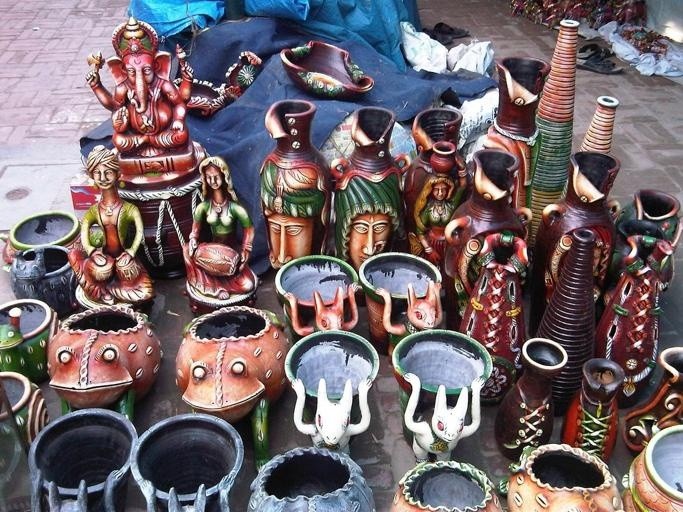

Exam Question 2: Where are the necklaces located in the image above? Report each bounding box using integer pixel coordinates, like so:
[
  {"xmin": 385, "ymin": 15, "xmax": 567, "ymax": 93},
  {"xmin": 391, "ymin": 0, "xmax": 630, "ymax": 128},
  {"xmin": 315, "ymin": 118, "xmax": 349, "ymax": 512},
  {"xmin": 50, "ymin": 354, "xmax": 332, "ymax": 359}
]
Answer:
[
  {"xmin": 100, "ymin": 199, "xmax": 120, "ymax": 216},
  {"xmin": 212, "ymin": 199, "xmax": 228, "ymax": 214}
]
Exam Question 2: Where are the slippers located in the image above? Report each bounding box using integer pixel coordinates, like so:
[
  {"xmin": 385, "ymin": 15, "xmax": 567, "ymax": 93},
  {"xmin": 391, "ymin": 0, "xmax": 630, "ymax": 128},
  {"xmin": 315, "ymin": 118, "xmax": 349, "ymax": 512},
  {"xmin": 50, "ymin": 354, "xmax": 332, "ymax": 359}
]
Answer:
[
  {"xmin": 422, "ymin": 22, "xmax": 470, "ymax": 45},
  {"xmin": 577, "ymin": 43, "xmax": 613, "ymax": 59},
  {"xmin": 575, "ymin": 55, "xmax": 623, "ymax": 75}
]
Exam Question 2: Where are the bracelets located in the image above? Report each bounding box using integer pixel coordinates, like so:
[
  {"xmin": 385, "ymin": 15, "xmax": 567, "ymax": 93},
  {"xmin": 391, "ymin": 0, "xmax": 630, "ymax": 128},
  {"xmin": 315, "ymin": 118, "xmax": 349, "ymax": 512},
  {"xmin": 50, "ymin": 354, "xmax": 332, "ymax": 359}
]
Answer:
[
  {"xmin": 457, "ymin": 167, "xmax": 467, "ymax": 177},
  {"xmin": 425, "ymin": 247, "xmax": 433, "ymax": 254}
]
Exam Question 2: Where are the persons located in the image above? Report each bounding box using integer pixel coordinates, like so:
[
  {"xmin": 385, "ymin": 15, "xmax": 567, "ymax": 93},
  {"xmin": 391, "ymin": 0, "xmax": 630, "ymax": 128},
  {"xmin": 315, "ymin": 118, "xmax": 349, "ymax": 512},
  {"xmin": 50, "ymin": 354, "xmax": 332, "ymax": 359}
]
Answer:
[
  {"xmin": 334, "ymin": 166, "xmax": 404, "ymax": 272},
  {"xmin": 413, "ymin": 154, "xmax": 467, "ymax": 298},
  {"xmin": 260, "ymin": 160, "xmax": 325, "ymax": 270},
  {"xmin": 68, "ymin": 145, "xmax": 155, "ymax": 306},
  {"xmin": 183, "ymin": 156, "xmax": 255, "ymax": 300}
]
